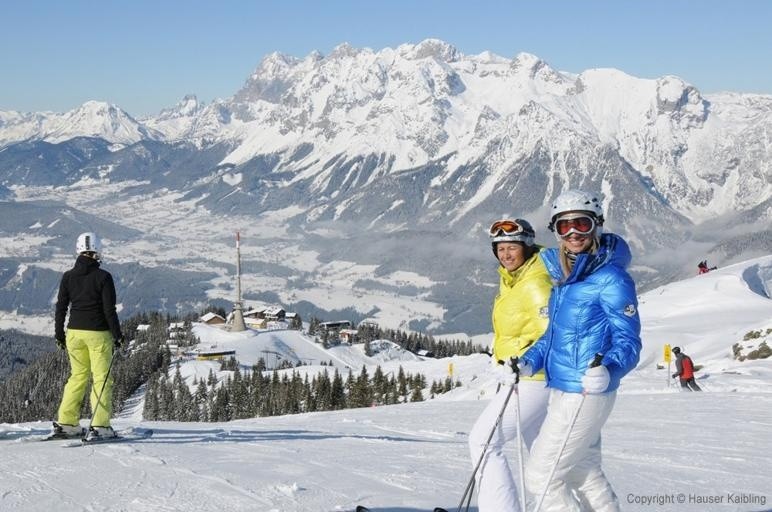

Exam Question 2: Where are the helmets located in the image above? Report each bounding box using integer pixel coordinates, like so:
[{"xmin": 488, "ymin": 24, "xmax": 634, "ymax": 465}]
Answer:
[
  {"xmin": 672, "ymin": 347, "xmax": 680, "ymax": 354},
  {"xmin": 76, "ymin": 231, "xmax": 101, "ymax": 257},
  {"xmin": 491, "ymin": 216, "xmax": 535, "ymax": 248},
  {"xmin": 549, "ymin": 188, "xmax": 603, "ymax": 225}
]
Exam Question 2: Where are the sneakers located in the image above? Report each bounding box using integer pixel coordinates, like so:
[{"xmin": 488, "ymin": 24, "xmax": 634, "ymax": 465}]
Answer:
[
  {"xmin": 58, "ymin": 423, "xmax": 83, "ymax": 437},
  {"xmin": 91, "ymin": 425, "xmax": 114, "ymax": 438}
]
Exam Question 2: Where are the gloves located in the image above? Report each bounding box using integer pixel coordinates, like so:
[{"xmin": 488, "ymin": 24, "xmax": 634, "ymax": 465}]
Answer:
[
  {"xmin": 505, "ymin": 359, "xmax": 532, "ymax": 384},
  {"xmin": 115, "ymin": 336, "xmax": 124, "ymax": 353},
  {"xmin": 581, "ymin": 365, "xmax": 612, "ymax": 394},
  {"xmin": 55, "ymin": 339, "xmax": 65, "ymax": 352}
]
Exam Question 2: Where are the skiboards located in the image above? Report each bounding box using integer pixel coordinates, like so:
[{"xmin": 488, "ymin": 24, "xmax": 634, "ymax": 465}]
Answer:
[{"xmin": 13, "ymin": 426, "xmax": 153, "ymax": 448}]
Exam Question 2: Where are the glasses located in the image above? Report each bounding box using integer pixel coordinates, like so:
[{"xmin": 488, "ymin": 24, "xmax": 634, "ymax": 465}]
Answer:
[
  {"xmin": 489, "ymin": 220, "xmax": 534, "ymax": 238},
  {"xmin": 549, "ymin": 215, "xmax": 603, "ymax": 239}
]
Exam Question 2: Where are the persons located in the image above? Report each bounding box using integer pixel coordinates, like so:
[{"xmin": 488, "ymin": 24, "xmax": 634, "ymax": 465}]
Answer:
[
  {"xmin": 467, "ymin": 219, "xmax": 566, "ymax": 510},
  {"xmin": 55, "ymin": 232, "xmax": 122, "ymax": 440},
  {"xmin": 505, "ymin": 189, "xmax": 640, "ymax": 512},
  {"xmin": 671, "ymin": 347, "xmax": 703, "ymax": 391},
  {"xmin": 703, "ymin": 260, "xmax": 708, "ymax": 274},
  {"xmin": 699, "ymin": 261, "xmax": 707, "ymax": 274}
]
